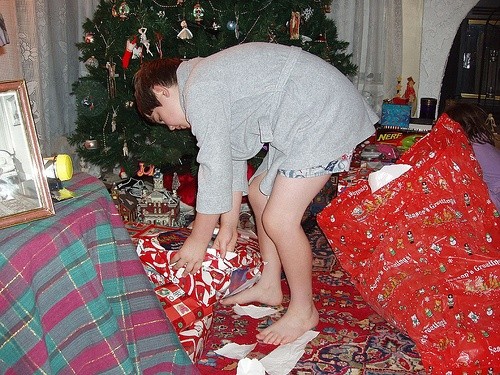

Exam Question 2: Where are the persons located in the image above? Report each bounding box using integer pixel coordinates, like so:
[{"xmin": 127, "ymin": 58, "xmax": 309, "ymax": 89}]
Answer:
[
  {"xmin": 132, "ymin": 41, "xmax": 380, "ymax": 346},
  {"xmin": 445, "ymin": 103, "xmax": 500, "ymax": 213}
]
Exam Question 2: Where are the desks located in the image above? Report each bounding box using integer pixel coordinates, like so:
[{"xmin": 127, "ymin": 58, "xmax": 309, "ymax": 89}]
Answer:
[{"xmin": 0, "ymin": 168, "xmax": 200, "ymax": 375}]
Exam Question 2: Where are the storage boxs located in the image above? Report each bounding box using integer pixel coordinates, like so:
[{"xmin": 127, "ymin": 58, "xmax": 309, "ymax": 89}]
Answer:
[{"xmin": 381, "ymin": 103, "xmax": 411, "ymax": 128}]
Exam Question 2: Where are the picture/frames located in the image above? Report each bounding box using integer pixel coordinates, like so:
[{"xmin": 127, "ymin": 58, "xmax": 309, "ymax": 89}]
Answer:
[{"xmin": 0, "ymin": 78, "xmax": 56, "ymax": 230}]
